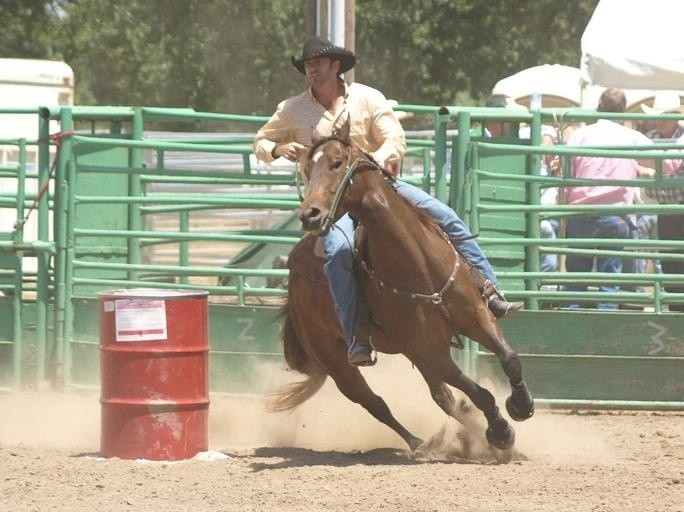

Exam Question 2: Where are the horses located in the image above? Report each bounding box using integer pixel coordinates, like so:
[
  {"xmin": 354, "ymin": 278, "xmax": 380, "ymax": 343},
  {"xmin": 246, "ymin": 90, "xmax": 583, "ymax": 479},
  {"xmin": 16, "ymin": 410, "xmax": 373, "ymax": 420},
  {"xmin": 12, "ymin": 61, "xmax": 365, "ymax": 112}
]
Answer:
[{"xmin": 256, "ymin": 110, "xmax": 535, "ymax": 464}]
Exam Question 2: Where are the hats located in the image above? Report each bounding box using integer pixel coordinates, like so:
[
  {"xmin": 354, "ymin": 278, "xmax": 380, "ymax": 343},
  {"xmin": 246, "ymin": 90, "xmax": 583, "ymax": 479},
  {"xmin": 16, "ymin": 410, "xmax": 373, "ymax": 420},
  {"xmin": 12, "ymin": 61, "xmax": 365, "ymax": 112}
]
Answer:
[
  {"xmin": 641, "ymin": 90, "xmax": 684, "ymax": 113},
  {"xmin": 291, "ymin": 36, "xmax": 356, "ymax": 76}
]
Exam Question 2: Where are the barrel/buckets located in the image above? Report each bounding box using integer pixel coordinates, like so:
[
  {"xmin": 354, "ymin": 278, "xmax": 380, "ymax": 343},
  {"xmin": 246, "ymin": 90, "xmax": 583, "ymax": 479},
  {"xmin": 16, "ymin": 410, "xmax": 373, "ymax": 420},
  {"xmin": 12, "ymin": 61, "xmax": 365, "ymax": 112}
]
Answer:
[{"xmin": 95, "ymin": 287, "xmax": 213, "ymax": 464}]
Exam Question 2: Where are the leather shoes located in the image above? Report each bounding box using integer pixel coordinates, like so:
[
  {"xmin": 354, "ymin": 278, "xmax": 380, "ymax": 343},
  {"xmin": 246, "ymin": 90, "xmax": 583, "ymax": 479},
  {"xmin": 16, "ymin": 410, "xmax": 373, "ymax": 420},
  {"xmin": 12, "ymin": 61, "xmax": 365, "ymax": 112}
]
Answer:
[
  {"xmin": 350, "ymin": 343, "xmax": 370, "ymax": 363},
  {"xmin": 488, "ymin": 297, "xmax": 524, "ymax": 319}
]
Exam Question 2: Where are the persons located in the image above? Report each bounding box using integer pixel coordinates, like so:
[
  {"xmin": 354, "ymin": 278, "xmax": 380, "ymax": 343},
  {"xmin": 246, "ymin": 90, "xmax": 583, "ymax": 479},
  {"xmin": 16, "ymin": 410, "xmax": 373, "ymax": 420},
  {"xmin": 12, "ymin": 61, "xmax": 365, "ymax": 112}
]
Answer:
[
  {"xmin": 449, "ymin": 88, "xmax": 684, "ymax": 316},
  {"xmin": 252, "ymin": 35, "xmax": 526, "ymax": 368}
]
